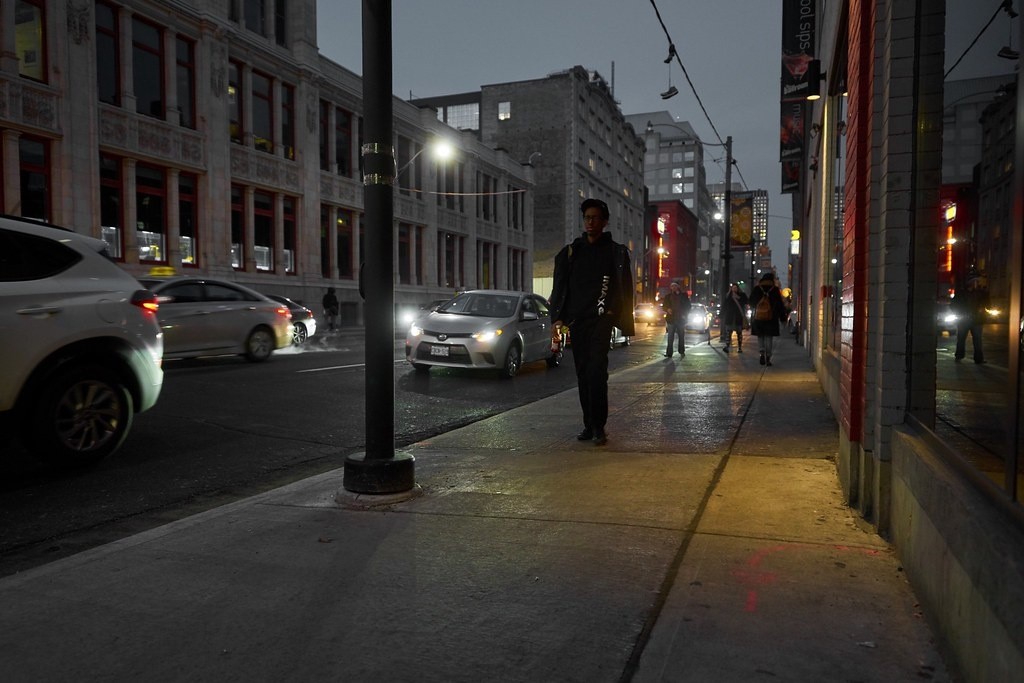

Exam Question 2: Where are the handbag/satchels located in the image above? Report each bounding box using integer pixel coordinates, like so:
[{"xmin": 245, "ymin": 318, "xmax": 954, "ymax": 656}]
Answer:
[
  {"xmin": 330, "ymin": 305, "xmax": 339, "ymax": 316},
  {"xmin": 743, "ymin": 316, "xmax": 750, "ymax": 330}
]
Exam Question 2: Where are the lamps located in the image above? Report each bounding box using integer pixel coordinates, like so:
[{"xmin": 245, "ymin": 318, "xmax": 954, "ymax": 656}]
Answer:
[
  {"xmin": 806, "ymin": 59, "xmax": 827, "ymax": 100},
  {"xmin": 809, "ymin": 123, "xmax": 821, "ymax": 138}
]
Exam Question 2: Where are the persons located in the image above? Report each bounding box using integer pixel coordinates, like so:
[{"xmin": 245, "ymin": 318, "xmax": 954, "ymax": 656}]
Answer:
[
  {"xmin": 719, "ymin": 283, "xmax": 749, "ymax": 353},
  {"xmin": 662, "ymin": 281, "xmax": 691, "ymax": 358},
  {"xmin": 548, "ymin": 199, "xmax": 637, "ymax": 446},
  {"xmin": 787, "ymin": 306, "xmax": 799, "ymax": 344},
  {"xmin": 953, "ymin": 253, "xmax": 988, "ymax": 366},
  {"xmin": 321, "ymin": 287, "xmax": 339, "ymax": 333},
  {"xmin": 749, "ymin": 272, "xmax": 785, "ymax": 366}
]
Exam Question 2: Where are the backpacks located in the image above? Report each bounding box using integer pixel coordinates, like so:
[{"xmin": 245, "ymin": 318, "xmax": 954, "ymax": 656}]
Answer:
[{"xmin": 754, "ymin": 285, "xmax": 776, "ymax": 321}]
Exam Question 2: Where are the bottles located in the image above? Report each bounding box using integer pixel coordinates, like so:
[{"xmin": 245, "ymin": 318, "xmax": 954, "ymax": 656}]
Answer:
[{"xmin": 551, "ymin": 325, "xmax": 562, "ymax": 351}]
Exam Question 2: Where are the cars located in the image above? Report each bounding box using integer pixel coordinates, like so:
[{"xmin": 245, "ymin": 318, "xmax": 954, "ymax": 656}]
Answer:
[
  {"xmin": 634, "ymin": 303, "xmax": 655, "ymax": 322},
  {"xmin": 565, "ymin": 326, "xmax": 631, "ymax": 349},
  {"xmin": 646, "ymin": 304, "xmax": 667, "ymax": 326},
  {"xmin": 136, "ymin": 277, "xmax": 293, "ymax": 365},
  {"xmin": 261, "ymin": 293, "xmax": 318, "ymax": 348},
  {"xmin": 406, "ymin": 291, "xmax": 566, "ymax": 379},
  {"xmin": 666, "ymin": 303, "xmax": 712, "ymax": 335},
  {"xmin": 936, "ymin": 304, "xmax": 958, "ymax": 340},
  {"xmin": 786, "ymin": 311, "xmax": 799, "ymax": 335},
  {"xmin": 707, "ymin": 307, "xmax": 720, "ymax": 328}
]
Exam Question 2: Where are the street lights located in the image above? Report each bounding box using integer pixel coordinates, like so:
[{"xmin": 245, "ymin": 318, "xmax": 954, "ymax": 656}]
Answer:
[{"xmin": 645, "ymin": 121, "xmax": 732, "ymax": 341}]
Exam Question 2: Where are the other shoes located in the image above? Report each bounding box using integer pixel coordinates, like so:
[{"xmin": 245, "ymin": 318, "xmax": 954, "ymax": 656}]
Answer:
[
  {"xmin": 766, "ymin": 358, "xmax": 772, "ymax": 366},
  {"xmin": 576, "ymin": 428, "xmax": 593, "ymax": 441},
  {"xmin": 592, "ymin": 431, "xmax": 607, "ymax": 444},
  {"xmin": 738, "ymin": 348, "xmax": 742, "ymax": 352},
  {"xmin": 760, "ymin": 356, "xmax": 765, "ymax": 366},
  {"xmin": 723, "ymin": 347, "xmax": 729, "ymax": 353}
]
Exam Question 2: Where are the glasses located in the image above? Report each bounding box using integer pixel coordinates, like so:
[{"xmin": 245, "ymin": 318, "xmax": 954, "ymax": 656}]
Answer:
[{"xmin": 583, "ymin": 215, "xmax": 602, "ymax": 222}]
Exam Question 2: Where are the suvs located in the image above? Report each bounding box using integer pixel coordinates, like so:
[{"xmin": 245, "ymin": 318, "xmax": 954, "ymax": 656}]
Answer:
[{"xmin": 0, "ymin": 212, "xmax": 168, "ymax": 474}]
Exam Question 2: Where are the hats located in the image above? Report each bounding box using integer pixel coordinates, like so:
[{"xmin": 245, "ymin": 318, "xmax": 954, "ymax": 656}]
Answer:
[{"xmin": 670, "ymin": 281, "xmax": 680, "ymax": 286}]
